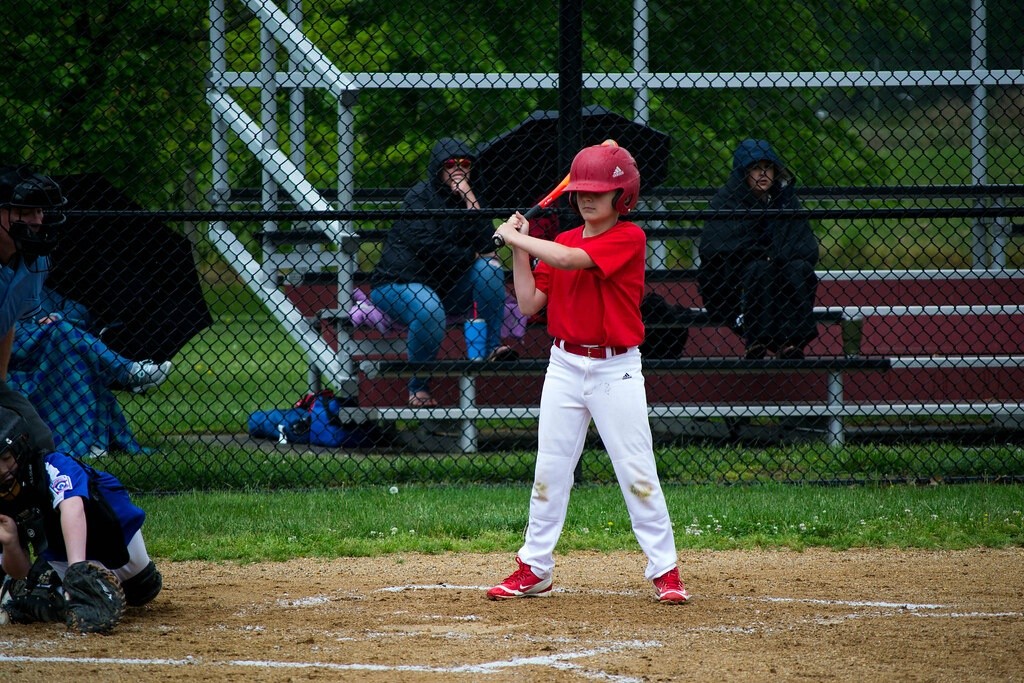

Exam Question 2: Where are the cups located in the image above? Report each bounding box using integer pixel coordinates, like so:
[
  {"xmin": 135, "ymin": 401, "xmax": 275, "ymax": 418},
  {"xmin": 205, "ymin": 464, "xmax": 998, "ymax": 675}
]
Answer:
[
  {"xmin": 464, "ymin": 319, "xmax": 486, "ymax": 361},
  {"xmin": 841, "ymin": 315, "xmax": 862, "ymax": 355}
]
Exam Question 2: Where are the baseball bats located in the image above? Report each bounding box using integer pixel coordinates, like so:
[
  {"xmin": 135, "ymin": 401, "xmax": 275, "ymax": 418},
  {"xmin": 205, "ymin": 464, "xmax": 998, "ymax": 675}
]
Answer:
[{"xmin": 491, "ymin": 139, "xmax": 618, "ymax": 249}]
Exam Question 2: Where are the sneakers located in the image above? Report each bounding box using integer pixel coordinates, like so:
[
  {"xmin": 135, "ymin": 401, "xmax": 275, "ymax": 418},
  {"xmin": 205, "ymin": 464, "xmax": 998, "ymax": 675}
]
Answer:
[
  {"xmin": 486, "ymin": 556, "xmax": 552, "ymax": 601},
  {"xmin": 130, "ymin": 360, "xmax": 172, "ymax": 393},
  {"xmin": 653, "ymin": 568, "xmax": 691, "ymax": 605}
]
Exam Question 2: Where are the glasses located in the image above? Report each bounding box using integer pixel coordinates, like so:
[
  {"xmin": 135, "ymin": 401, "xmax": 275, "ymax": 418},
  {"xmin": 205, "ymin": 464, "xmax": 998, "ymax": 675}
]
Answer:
[{"xmin": 442, "ymin": 158, "xmax": 473, "ymax": 169}]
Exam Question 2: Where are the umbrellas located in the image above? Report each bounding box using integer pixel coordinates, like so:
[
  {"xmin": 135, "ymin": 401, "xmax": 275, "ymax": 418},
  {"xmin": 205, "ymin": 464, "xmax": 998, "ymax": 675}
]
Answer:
[
  {"xmin": 0, "ymin": 174, "xmax": 214, "ymax": 366},
  {"xmin": 471, "ymin": 107, "xmax": 673, "ymax": 232}
]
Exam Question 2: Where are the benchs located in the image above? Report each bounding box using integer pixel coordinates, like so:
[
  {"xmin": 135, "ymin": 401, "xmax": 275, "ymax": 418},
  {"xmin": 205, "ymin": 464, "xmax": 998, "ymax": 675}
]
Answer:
[{"xmin": 211, "ymin": 184, "xmax": 892, "ymax": 448}]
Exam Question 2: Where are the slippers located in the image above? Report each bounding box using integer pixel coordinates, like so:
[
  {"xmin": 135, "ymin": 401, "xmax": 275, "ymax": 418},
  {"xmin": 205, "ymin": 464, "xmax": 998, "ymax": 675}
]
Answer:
[
  {"xmin": 409, "ymin": 392, "xmax": 435, "ymax": 408},
  {"xmin": 490, "ymin": 348, "xmax": 518, "ymax": 364}
]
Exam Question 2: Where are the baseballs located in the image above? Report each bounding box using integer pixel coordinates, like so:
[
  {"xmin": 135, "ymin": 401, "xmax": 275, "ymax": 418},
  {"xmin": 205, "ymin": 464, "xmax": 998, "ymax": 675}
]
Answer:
[{"xmin": 0, "ymin": 608, "xmax": 9, "ymax": 625}]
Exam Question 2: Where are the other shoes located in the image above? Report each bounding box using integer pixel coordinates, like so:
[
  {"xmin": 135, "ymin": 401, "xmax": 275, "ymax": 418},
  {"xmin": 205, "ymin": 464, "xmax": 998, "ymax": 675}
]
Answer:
[
  {"xmin": 782, "ymin": 346, "xmax": 805, "ymax": 358},
  {"xmin": 744, "ymin": 345, "xmax": 767, "ymax": 359}
]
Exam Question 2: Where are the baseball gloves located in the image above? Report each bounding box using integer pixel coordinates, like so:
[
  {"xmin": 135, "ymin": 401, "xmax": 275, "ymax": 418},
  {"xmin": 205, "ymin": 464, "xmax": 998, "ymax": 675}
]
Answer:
[{"xmin": 62, "ymin": 560, "xmax": 127, "ymax": 634}]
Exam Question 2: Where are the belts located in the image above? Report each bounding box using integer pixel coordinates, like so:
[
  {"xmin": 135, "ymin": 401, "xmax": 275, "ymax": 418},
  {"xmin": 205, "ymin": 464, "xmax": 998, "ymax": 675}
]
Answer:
[{"xmin": 554, "ymin": 337, "xmax": 627, "ymax": 360}]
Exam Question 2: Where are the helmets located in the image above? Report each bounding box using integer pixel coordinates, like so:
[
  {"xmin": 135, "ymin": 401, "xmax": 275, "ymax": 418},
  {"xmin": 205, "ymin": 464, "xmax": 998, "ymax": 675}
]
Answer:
[
  {"xmin": 562, "ymin": 145, "xmax": 641, "ymax": 216},
  {"xmin": 0, "ymin": 407, "xmax": 30, "ymax": 497}
]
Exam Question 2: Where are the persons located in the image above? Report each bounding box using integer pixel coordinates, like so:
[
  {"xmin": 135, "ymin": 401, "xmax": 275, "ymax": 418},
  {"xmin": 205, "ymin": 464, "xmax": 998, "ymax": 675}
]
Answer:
[
  {"xmin": 699, "ymin": 139, "xmax": 821, "ymax": 360},
  {"xmin": 0, "ymin": 169, "xmax": 173, "ymax": 631},
  {"xmin": 486, "ymin": 145, "xmax": 687, "ymax": 604},
  {"xmin": 523, "ymin": 192, "xmax": 578, "ymax": 323},
  {"xmin": 368, "ymin": 136, "xmax": 517, "ymax": 408}
]
